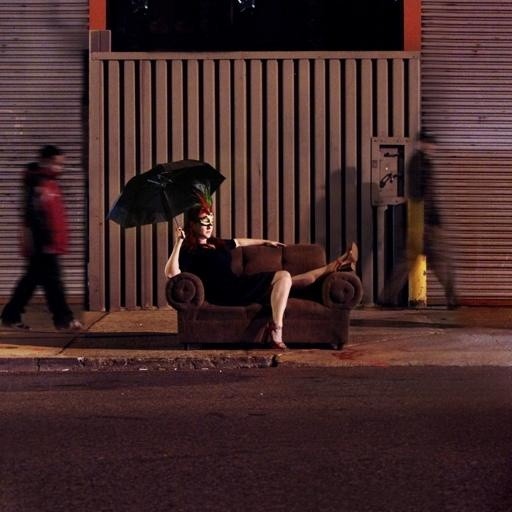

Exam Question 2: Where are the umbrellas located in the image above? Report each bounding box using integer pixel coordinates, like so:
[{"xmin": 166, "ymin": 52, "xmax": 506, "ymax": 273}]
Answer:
[{"xmin": 107, "ymin": 160, "xmax": 226, "ymax": 239}]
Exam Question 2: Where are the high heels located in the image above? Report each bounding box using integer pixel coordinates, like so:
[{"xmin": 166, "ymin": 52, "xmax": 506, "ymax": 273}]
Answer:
[
  {"xmin": 254, "ymin": 318, "xmax": 288, "ymax": 350},
  {"xmin": 334, "ymin": 239, "xmax": 360, "ymax": 273}
]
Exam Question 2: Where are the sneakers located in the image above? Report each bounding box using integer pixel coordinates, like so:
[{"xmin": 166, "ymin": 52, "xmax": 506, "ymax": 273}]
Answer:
[
  {"xmin": 0, "ymin": 320, "xmax": 33, "ymax": 332},
  {"xmin": 56, "ymin": 319, "xmax": 86, "ymax": 330}
]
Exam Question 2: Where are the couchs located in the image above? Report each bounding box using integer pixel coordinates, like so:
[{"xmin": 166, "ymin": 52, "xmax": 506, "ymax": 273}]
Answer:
[{"xmin": 165, "ymin": 244, "xmax": 363, "ymax": 352}]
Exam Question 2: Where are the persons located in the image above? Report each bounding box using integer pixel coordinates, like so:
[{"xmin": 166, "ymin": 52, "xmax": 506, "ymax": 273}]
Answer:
[
  {"xmin": 164, "ymin": 204, "xmax": 358, "ymax": 349},
  {"xmin": 377, "ymin": 130, "xmax": 464, "ymax": 310},
  {"xmin": 0, "ymin": 144, "xmax": 83, "ymax": 330}
]
